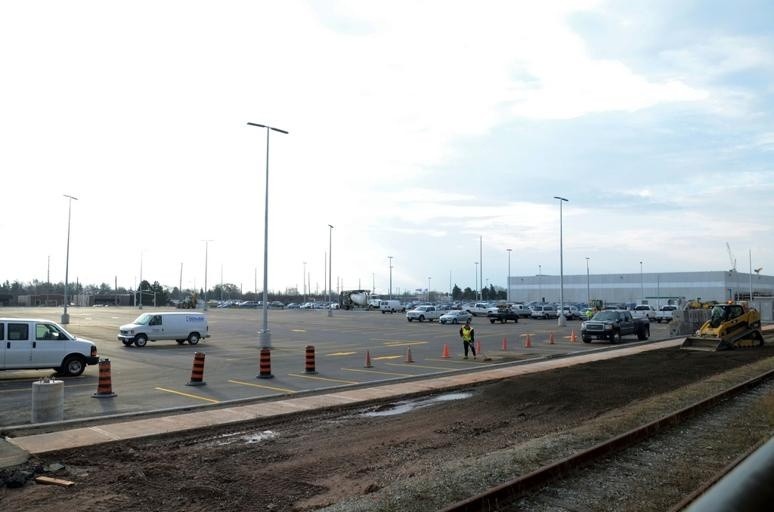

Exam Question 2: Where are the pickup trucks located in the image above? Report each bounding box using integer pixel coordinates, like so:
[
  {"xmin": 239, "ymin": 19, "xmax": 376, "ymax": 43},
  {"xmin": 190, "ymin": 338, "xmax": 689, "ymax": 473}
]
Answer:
[
  {"xmin": 628, "ymin": 304, "xmax": 657, "ymax": 321},
  {"xmin": 578, "ymin": 309, "xmax": 652, "ymax": 345},
  {"xmin": 652, "ymin": 304, "xmax": 682, "ymax": 323},
  {"xmin": 405, "ymin": 303, "xmax": 446, "ymax": 323}
]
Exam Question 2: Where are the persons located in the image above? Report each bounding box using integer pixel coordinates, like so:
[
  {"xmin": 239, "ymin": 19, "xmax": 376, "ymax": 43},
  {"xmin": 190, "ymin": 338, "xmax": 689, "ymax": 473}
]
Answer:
[{"xmin": 460, "ymin": 320, "xmax": 476, "ymax": 360}]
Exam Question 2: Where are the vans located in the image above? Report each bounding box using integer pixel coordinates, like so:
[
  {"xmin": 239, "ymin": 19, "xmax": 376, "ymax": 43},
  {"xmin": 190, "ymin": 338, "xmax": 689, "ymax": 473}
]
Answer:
[{"xmin": 0, "ymin": 318, "xmax": 102, "ymax": 380}]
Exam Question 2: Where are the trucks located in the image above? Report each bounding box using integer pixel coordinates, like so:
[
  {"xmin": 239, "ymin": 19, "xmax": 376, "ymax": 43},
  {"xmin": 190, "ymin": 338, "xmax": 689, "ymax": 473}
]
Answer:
[{"xmin": 119, "ymin": 311, "xmax": 210, "ymax": 347}]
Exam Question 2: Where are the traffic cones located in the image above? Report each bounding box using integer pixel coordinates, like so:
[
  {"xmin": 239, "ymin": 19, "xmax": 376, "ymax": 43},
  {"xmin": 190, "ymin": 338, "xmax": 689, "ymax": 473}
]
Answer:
[
  {"xmin": 523, "ymin": 335, "xmax": 533, "ymax": 349},
  {"xmin": 501, "ymin": 335, "xmax": 509, "ymax": 352},
  {"xmin": 569, "ymin": 329, "xmax": 577, "ymax": 341},
  {"xmin": 548, "ymin": 331, "xmax": 554, "ymax": 345},
  {"xmin": 476, "ymin": 340, "xmax": 481, "ymax": 355},
  {"xmin": 362, "ymin": 350, "xmax": 374, "ymax": 369},
  {"xmin": 404, "ymin": 343, "xmax": 416, "ymax": 364},
  {"xmin": 439, "ymin": 343, "xmax": 452, "ymax": 358}
]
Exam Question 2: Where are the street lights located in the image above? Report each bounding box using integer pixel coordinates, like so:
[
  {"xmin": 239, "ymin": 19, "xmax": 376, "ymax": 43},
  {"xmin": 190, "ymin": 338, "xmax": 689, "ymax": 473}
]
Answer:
[
  {"xmin": 554, "ymin": 195, "xmax": 570, "ymax": 327},
  {"xmin": 59, "ymin": 193, "xmax": 81, "ymax": 323},
  {"xmin": 474, "ymin": 260, "xmax": 480, "ymax": 301},
  {"xmin": 327, "ymin": 221, "xmax": 337, "ymax": 316},
  {"xmin": 426, "ymin": 276, "xmax": 434, "ymax": 303},
  {"xmin": 387, "ymin": 254, "xmax": 395, "ymax": 302},
  {"xmin": 505, "ymin": 247, "xmax": 513, "ymax": 302},
  {"xmin": 246, "ymin": 120, "xmax": 290, "ymax": 350},
  {"xmin": 585, "ymin": 257, "xmax": 592, "ymax": 304},
  {"xmin": 639, "ymin": 259, "xmax": 644, "ymax": 300}
]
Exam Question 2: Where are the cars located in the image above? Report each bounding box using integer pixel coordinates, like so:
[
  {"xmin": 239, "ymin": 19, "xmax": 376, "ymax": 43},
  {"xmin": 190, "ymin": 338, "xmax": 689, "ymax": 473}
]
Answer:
[
  {"xmin": 438, "ymin": 308, "xmax": 475, "ymax": 325},
  {"xmin": 486, "ymin": 303, "xmax": 521, "ymax": 325}
]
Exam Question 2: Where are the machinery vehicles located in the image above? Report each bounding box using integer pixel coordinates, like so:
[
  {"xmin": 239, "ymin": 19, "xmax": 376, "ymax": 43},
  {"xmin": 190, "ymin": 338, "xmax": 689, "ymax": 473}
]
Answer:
[
  {"xmin": 667, "ymin": 297, "xmax": 719, "ymax": 337},
  {"xmin": 339, "ymin": 290, "xmax": 382, "ymax": 311},
  {"xmin": 680, "ymin": 299, "xmax": 767, "ymax": 354}
]
